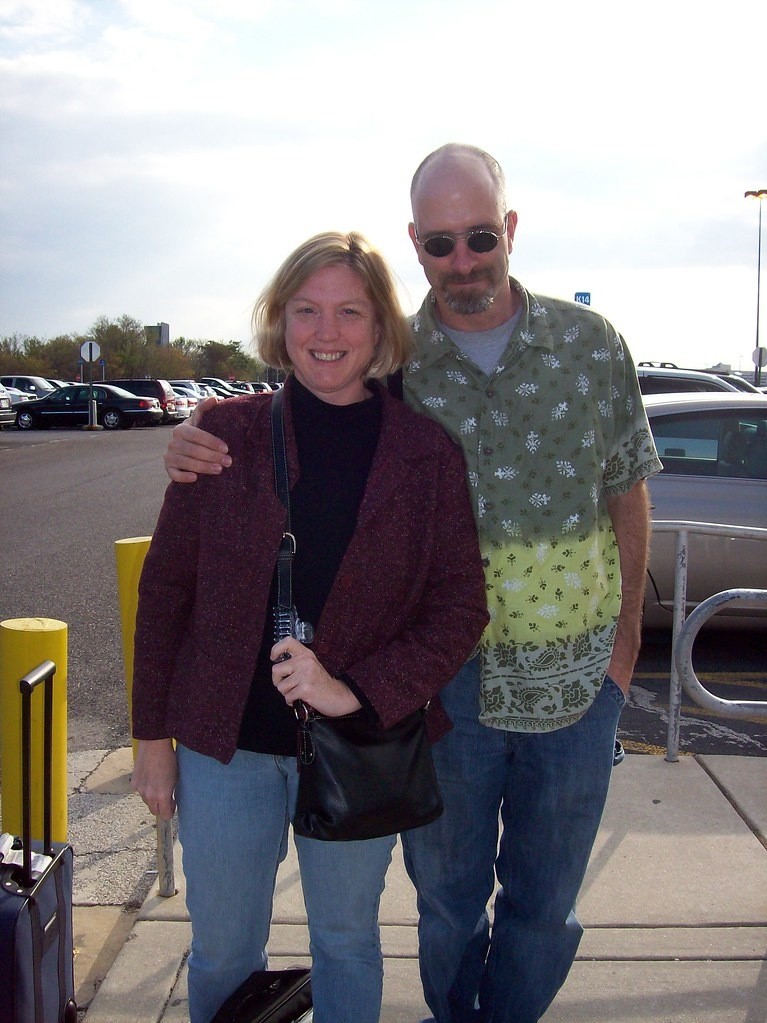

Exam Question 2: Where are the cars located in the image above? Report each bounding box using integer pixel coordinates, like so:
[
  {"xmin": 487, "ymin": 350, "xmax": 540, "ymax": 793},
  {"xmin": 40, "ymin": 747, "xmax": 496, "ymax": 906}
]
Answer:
[
  {"xmin": 171, "ymin": 386, "xmax": 205, "ymax": 404},
  {"xmin": 46, "ymin": 378, "xmax": 86, "ymax": 389},
  {"xmin": 174, "ymin": 391, "xmax": 198, "ymax": 413},
  {"xmin": 4, "ymin": 386, "xmax": 37, "ymax": 402},
  {"xmin": 267, "ymin": 382, "xmax": 284, "ymax": 390},
  {"xmin": 0, "ymin": 383, "xmax": 16, "ymax": 428},
  {"xmin": 12, "ymin": 385, "xmax": 163, "ymax": 431},
  {"xmin": 628, "ymin": 392, "xmax": 767, "ymax": 643}
]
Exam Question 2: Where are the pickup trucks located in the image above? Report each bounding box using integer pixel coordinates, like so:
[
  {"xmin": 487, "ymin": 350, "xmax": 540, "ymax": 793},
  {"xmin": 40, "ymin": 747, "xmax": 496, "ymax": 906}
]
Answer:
[{"xmin": 198, "ymin": 377, "xmax": 249, "ymax": 399}]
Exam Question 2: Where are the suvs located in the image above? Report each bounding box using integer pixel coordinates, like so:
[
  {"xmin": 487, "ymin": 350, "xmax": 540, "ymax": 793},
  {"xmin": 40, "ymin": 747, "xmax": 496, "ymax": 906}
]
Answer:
[{"xmin": 635, "ymin": 361, "xmax": 767, "ymax": 440}]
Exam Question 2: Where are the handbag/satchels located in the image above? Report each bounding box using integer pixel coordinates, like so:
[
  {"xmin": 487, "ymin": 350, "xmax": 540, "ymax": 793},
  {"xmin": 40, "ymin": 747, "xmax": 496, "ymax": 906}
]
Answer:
[
  {"xmin": 292, "ymin": 708, "xmax": 442, "ymax": 841},
  {"xmin": 207, "ymin": 969, "xmax": 315, "ymax": 1023}
]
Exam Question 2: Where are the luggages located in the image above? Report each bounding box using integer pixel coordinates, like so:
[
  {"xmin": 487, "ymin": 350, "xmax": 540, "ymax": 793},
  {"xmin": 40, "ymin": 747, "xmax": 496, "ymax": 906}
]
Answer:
[{"xmin": 0, "ymin": 660, "xmax": 76, "ymax": 1023}]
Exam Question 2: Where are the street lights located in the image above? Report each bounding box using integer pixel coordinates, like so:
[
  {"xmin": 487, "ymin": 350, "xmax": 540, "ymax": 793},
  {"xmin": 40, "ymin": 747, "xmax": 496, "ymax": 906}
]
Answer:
[{"xmin": 744, "ymin": 189, "xmax": 767, "ymax": 387}]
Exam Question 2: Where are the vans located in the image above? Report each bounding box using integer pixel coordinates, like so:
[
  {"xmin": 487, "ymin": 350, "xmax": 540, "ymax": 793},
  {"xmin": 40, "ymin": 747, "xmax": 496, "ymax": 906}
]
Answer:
[
  {"xmin": 0, "ymin": 375, "xmax": 54, "ymax": 397},
  {"xmin": 226, "ymin": 380, "xmax": 255, "ymax": 393},
  {"xmin": 93, "ymin": 378, "xmax": 178, "ymax": 423},
  {"xmin": 250, "ymin": 382, "xmax": 272, "ymax": 393},
  {"xmin": 166, "ymin": 380, "xmax": 201, "ymax": 394}
]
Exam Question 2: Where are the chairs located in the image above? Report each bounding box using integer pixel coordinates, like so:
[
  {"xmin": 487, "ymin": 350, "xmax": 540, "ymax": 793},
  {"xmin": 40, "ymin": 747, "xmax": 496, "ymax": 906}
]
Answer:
[{"xmin": 716, "ymin": 420, "xmax": 767, "ymax": 480}]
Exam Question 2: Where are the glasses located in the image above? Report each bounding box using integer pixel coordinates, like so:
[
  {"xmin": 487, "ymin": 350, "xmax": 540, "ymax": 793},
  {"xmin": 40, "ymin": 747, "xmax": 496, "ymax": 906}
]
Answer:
[{"xmin": 414, "ymin": 214, "xmax": 510, "ymax": 257}]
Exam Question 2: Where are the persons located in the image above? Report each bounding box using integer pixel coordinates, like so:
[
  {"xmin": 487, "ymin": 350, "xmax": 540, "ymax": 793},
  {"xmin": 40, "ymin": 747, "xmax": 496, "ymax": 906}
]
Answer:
[
  {"xmin": 160, "ymin": 141, "xmax": 666, "ymax": 1023},
  {"xmin": 131, "ymin": 230, "xmax": 491, "ymax": 1023}
]
